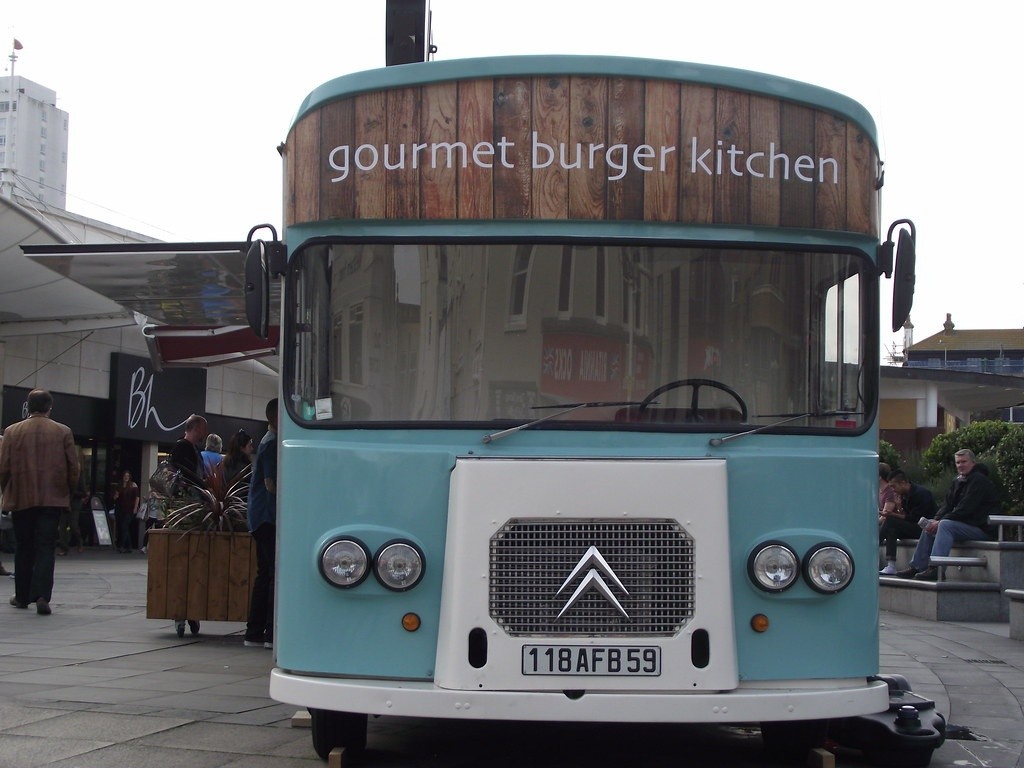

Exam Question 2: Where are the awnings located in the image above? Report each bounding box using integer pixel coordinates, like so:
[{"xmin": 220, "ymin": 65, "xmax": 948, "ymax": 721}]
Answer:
[{"xmin": 0, "ymin": 190, "xmax": 140, "ymax": 341}]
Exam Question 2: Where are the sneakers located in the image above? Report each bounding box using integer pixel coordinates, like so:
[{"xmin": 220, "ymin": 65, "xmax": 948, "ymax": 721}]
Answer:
[{"xmin": 896, "ymin": 567, "xmax": 946, "ymax": 580}]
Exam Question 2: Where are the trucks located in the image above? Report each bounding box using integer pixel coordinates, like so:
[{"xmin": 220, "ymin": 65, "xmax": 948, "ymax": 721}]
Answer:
[{"xmin": 12, "ymin": 52, "xmax": 920, "ymax": 765}]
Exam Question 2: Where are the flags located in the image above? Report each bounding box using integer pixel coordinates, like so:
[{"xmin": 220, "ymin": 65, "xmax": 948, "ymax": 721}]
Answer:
[{"xmin": 13, "ymin": 39, "xmax": 23, "ymax": 50}]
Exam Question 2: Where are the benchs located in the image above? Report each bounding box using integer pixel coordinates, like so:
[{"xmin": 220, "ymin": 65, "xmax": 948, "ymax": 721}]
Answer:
[{"xmin": 879, "ymin": 514, "xmax": 1024, "ymax": 641}]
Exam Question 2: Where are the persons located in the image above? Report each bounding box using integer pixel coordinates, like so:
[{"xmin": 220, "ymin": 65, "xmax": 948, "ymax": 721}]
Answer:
[
  {"xmin": 138, "ymin": 488, "xmax": 170, "ymax": 555},
  {"xmin": 200, "ymin": 433, "xmax": 227, "ymax": 479},
  {"xmin": 895, "ymin": 449, "xmax": 1000, "ymax": 581},
  {"xmin": 876, "ymin": 463, "xmax": 902, "ymax": 534},
  {"xmin": 872, "ymin": 470, "xmax": 940, "ymax": 575},
  {"xmin": 168, "ymin": 414, "xmax": 207, "ymax": 501},
  {"xmin": 111, "ymin": 472, "xmax": 140, "ymax": 554},
  {"xmin": 218, "ymin": 427, "xmax": 255, "ymax": 515},
  {"xmin": 1, "ymin": 388, "xmax": 81, "ymax": 616},
  {"xmin": 242, "ymin": 398, "xmax": 280, "ymax": 649}
]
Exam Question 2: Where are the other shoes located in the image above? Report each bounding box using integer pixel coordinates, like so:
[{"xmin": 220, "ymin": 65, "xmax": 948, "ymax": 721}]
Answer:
[
  {"xmin": 141, "ymin": 547, "xmax": 147, "ymax": 554},
  {"xmin": 0, "ymin": 566, "xmax": 10, "ymax": 577},
  {"xmin": 243, "ymin": 628, "xmax": 272, "ymax": 650},
  {"xmin": 117, "ymin": 547, "xmax": 133, "ymax": 554},
  {"xmin": 880, "ymin": 566, "xmax": 897, "ymax": 575},
  {"xmin": 9, "ymin": 594, "xmax": 52, "ymax": 616}
]
